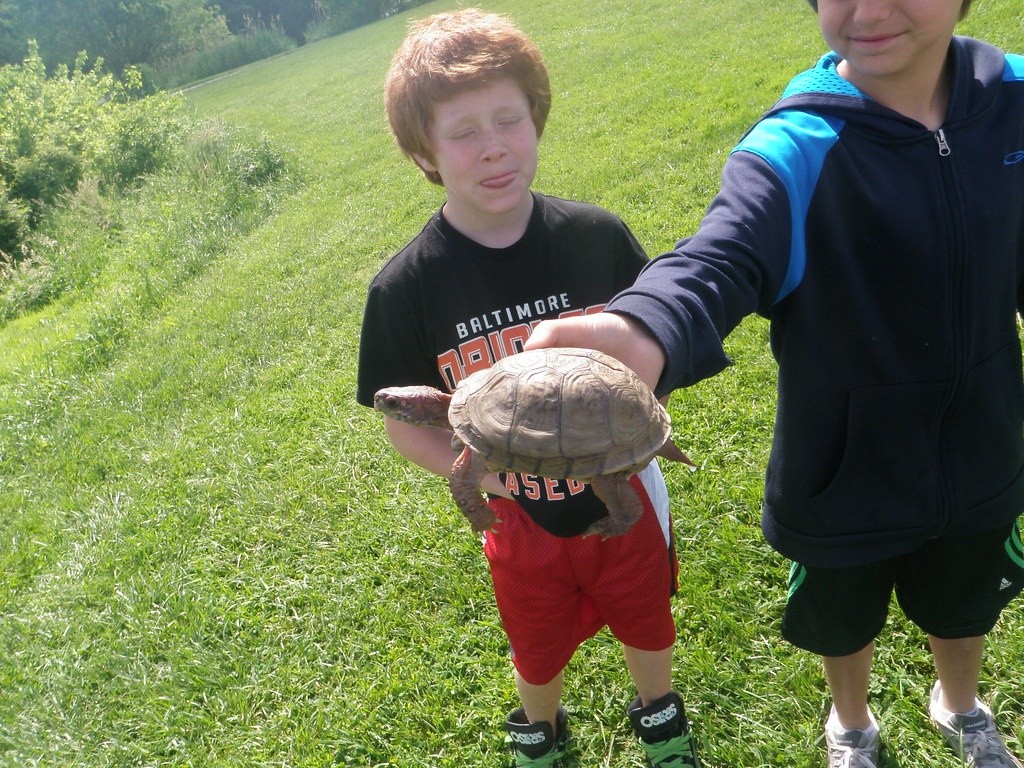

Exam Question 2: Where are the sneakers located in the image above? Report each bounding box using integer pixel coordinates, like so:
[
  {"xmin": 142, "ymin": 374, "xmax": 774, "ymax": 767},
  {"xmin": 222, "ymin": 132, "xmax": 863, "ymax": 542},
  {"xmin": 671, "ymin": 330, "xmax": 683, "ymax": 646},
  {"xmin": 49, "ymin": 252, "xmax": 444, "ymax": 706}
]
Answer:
[
  {"xmin": 930, "ymin": 679, "xmax": 1023, "ymax": 768},
  {"xmin": 825, "ymin": 703, "xmax": 880, "ymax": 768},
  {"xmin": 627, "ymin": 690, "xmax": 704, "ymax": 768},
  {"xmin": 505, "ymin": 706, "xmax": 567, "ymax": 768}
]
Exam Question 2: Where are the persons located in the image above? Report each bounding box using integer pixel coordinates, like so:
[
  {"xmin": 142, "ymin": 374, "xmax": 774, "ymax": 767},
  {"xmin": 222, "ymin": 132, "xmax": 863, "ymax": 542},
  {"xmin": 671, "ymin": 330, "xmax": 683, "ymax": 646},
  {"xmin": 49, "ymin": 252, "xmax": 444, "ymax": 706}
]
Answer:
[
  {"xmin": 524, "ymin": 0, "xmax": 1023, "ymax": 768},
  {"xmin": 357, "ymin": 10, "xmax": 702, "ymax": 768}
]
